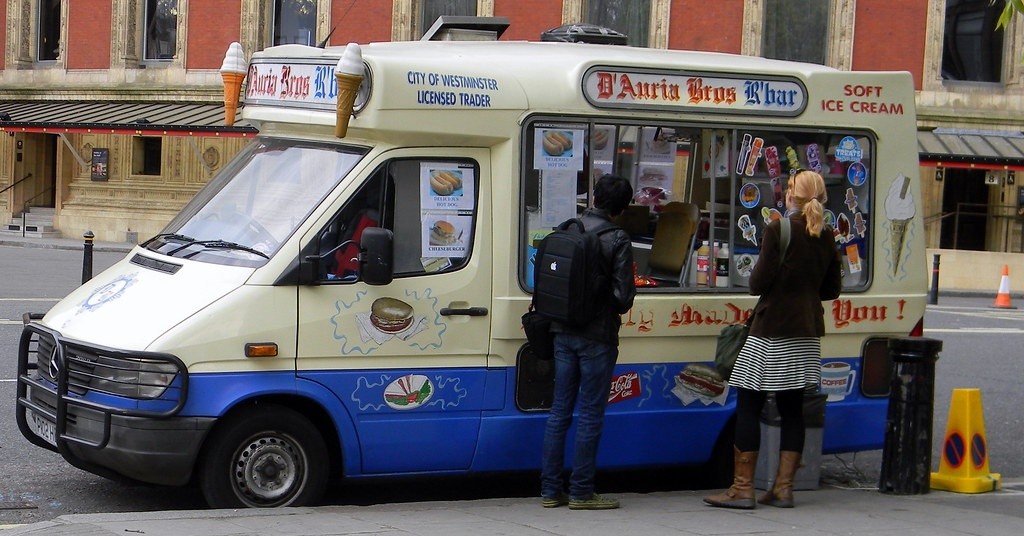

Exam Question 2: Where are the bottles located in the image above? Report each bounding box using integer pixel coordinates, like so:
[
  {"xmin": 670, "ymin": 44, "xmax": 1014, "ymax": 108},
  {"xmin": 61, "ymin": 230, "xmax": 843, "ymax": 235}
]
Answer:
[
  {"xmin": 712, "ymin": 242, "xmax": 720, "ymax": 286},
  {"xmin": 716, "ymin": 243, "xmax": 729, "ymax": 288},
  {"xmin": 697, "ymin": 241, "xmax": 710, "ymax": 284}
]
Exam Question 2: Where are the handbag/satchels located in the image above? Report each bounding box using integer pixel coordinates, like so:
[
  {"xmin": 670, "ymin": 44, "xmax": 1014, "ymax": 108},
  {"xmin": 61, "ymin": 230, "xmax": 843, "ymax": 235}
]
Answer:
[
  {"xmin": 522, "ymin": 304, "xmax": 552, "ymax": 352},
  {"xmin": 715, "ymin": 324, "xmax": 750, "ymax": 380}
]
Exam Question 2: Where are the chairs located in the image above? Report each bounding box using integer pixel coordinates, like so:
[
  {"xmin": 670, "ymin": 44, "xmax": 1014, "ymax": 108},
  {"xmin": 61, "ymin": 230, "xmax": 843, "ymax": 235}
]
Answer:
[{"xmin": 644, "ymin": 203, "xmax": 703, "ymax": 288}]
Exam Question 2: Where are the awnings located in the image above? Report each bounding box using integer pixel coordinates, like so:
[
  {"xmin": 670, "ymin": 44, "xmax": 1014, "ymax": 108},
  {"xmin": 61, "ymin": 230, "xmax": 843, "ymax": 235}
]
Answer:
[
  {"xmin": 618, "ymin": 124, "xmax": 1023, "ymax": 171},
  {"xmin": 0, "ymin": 98, "xmax": 262, "ymax": 167}
]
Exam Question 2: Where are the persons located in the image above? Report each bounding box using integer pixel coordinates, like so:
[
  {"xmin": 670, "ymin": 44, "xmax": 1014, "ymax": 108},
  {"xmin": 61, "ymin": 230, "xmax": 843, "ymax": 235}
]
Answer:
[
  {"xmin": 539, "ymin": 174, "xmax": 637, "ymax": 510},
  {"xmin": 702, "ymin": 169, "xmax": 842, "ymax": 508}
]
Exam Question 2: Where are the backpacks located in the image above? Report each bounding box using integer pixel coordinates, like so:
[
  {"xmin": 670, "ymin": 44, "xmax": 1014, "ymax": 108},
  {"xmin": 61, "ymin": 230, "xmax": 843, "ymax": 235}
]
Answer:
[{"xmin": 532, "ymin": 218, "xmax": 625, "ymax": 322}]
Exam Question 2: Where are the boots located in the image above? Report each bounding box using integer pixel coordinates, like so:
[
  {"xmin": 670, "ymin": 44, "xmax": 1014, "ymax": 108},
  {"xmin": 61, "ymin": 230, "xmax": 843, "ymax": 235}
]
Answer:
[
  {"xmin": 703, "ymin": 444, "xmax": 759, "ymax": 508},
  {"xmin": 757, "ymin": 450, "xmax": 801, "ymax": 508}
]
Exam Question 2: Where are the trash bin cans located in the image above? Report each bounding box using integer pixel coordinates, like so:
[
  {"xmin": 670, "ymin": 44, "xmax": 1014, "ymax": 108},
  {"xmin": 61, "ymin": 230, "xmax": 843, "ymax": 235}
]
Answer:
[{"xmin": 753, "ymin": 391, "xmax": 829, "ymax": 490}]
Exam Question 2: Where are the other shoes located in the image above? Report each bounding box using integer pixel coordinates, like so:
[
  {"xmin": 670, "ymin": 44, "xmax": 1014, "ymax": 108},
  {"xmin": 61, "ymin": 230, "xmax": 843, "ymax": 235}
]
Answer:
[
  {"xmin": 569, "ymin": 493, "xmax": 619, "ymax": 510},
  {"xmin": 543, "ymin": 495, "xmax": 568, "ymax": 508}
]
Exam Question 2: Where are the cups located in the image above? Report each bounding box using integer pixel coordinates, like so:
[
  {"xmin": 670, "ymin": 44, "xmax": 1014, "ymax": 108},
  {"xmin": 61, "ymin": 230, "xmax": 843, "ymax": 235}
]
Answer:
[{"xmin": 820, "ymin": 362, "xmax": 856, "ymax": 402}]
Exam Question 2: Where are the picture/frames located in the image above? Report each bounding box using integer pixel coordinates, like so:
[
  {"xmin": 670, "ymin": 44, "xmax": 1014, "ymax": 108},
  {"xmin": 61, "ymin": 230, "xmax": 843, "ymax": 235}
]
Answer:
[{"xmin": 91, "ymin": 146, "xmax": 109, "ymax": 180}]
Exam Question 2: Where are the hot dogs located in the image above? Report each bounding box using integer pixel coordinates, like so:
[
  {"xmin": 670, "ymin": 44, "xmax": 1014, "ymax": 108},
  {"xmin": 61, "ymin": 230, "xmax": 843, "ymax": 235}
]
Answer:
[
  {"xmin": 543, "ymin": 131, "xmax": 574, "ymax": 156},
  {"xmin": 430, "ymin": 170, "xmax": 462, "ymax": 196},
  {"xmin": 594, "ymin": 131, "xmax": 608, "ymax": 150}
]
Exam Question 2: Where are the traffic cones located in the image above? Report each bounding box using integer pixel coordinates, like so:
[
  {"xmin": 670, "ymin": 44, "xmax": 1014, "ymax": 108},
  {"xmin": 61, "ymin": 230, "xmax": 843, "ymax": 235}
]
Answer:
[{"xmin": 990, "ymin": 264, "xmax": 1018, "ymax": 309}]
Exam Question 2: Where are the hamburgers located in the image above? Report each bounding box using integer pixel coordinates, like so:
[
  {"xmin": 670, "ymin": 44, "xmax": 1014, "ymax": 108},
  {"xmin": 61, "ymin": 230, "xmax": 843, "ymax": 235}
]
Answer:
[
  {"xmin": 640, "ymin": 167, "xmax": 668, "ymax": 184},
  {"xmin": 679, "ymin": 364, "xmax": 725, "ymax": 396},
  {"xmin": 431, "ymin": 220, "xmax": 456, "ymax": 243}
]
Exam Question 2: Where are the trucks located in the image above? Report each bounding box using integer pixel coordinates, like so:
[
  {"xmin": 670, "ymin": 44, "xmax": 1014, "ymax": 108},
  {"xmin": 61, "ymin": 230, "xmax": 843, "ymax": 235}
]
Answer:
[{"xmin": 14, "ymin": 40, "xmax": 930, "ymax": 508}]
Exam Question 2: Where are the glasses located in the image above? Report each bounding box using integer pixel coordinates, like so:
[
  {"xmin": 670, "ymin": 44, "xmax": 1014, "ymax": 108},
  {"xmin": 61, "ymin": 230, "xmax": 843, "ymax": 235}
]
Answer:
[{"xmin": 794, "ymin": 168, "xmax": 806, "ymax": 185}]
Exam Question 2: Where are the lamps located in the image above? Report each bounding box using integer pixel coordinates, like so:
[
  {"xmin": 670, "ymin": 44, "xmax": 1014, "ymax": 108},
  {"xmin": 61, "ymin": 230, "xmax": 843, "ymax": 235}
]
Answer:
[
  {"xmin": 0, "ymin": 112, "xmax": 11, "ymax": 121},
  {"xmin": 136, "ymin": 118, "xmax": 150, "ymax": 124}
]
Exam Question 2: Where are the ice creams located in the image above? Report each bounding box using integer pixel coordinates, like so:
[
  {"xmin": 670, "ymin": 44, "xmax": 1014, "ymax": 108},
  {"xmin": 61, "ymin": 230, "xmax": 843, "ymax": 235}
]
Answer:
[
  {"xmin": 885, "ymin": 172, "xmax": 916, "ymax": 277},
  {"xmin": 334, "ymin": 43, "xmax": 364, "ymax": 138},
  {"xmin": 219, "ymin": 42, "xmax": 248, "ymax": 126}
]
